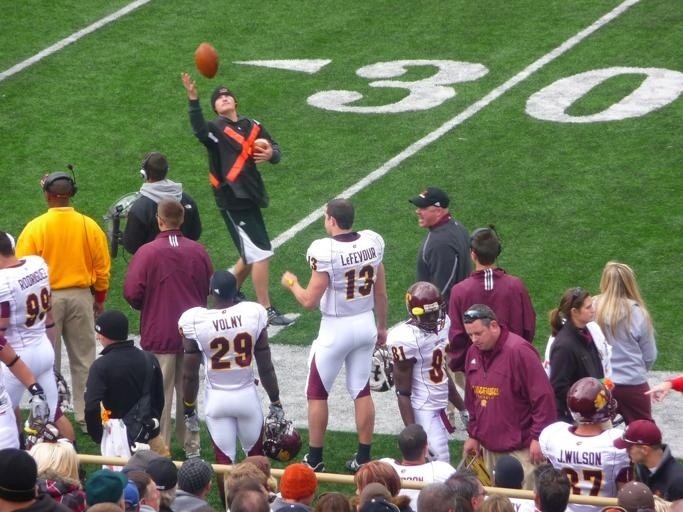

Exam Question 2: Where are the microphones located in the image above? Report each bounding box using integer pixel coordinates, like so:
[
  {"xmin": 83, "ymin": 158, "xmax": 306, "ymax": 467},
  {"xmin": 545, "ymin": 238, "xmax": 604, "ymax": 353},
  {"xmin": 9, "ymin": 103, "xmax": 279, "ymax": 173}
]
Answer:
[
  {"xmin": 67, "ymin": 163, "xmax": 75, "ymax": 184},
  {"xmin": 489, "ymin": 223, "xmax": 499, "ymax": 237}
]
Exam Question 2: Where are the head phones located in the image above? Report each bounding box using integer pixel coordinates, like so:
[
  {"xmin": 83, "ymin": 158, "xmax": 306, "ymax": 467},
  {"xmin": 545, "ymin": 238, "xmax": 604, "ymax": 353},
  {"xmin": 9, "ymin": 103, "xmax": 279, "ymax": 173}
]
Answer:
[
  {"xmin": 469, "ymin": 228, "xmax": 502, "ymax": 256},
  {"xmin": 43, "ymin": 172, "xmax": 78, "ymax": 196},
  {"xmin": 140, "ymin": 152, "xmax": 155, "ymax": 180}
]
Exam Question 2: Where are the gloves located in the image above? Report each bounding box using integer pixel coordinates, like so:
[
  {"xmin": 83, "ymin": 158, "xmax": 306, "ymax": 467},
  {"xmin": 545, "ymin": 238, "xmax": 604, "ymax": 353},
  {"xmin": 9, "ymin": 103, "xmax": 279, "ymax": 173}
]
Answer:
[
  {"xmin": 29, "ymin": 384, "xmax": 49, "ymax": 418},
  {"xmin": 184, "ymin": 402, "xmax": 200, "ymax": 432}
]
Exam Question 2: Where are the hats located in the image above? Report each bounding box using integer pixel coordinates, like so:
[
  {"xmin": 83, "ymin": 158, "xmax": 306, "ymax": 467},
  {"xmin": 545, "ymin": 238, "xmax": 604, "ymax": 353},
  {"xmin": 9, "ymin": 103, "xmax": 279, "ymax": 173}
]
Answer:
[
  {"xmin": 86, "ymin": 458, "xmax": 212, "ymax": 505},
  {"xmin": 211, "ymin": 271, "xmax": 236, "ymax": 298},
  {"xmin": 281, "ymin": 463, "xmax": 316, "ymax": 500},
  {"xmin": 94, "ymin": 310, "xmax": 128, "ymax": 340},
  {"xmin": 409, "ymin": 188, "xmax": 449, "ymax": 207},
  {"xmin": 0, "ymin": 448, "xmax": 36, "ymax": 500},
  {"xmin": 614, "ymin": 420, "xmax": 661, "ymax": 449},
  {"xmin": 212, "ymin": 87, "xmax": 236, "ymax": 108}
]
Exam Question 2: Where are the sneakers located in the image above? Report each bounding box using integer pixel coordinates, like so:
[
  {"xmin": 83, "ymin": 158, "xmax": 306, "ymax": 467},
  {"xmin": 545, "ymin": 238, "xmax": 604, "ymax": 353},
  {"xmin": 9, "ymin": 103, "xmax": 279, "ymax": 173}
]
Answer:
[
  {"xmin": 302, "ymin": 454, "xmax": 325, "ymax": 472},
  {"xmin": 346, "ymin": 459, "xmax": 363, "ymax": 471},
  {"xmin": 267, "ymin": 306, "xmax": 294, "ymax": 326}
]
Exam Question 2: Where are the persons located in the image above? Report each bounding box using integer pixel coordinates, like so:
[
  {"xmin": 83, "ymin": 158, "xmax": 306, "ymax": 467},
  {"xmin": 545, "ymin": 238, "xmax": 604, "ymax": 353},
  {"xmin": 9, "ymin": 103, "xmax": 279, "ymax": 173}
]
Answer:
[
  {"xmin": 181, "ymin": 70, "xmax": 295, "ymax": 326},
  {"xmin": 1, "ymin": 152, "xmax": 683, "ymax": 512}
]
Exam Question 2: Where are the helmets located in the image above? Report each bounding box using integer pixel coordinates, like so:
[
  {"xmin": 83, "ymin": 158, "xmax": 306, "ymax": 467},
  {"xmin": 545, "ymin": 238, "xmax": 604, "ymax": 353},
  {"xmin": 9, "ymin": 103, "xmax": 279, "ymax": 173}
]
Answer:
[
  {"xmin": 24, "ymin": 421, "xmax": 59, "ymax": 449},
  {"xmin": 567, "ymin": 377, "xmax": 617, "ymax": 423},
  {"xmin": 264, "ymin": 416, "xmax": 301, "ymax": 461},
  {"xmin": 369, "ymin": 346, "xmax": 393, "ymax": 391},
  {"xmin": 405, "ymin": 281, "xmax": 446, "ymax": 331}
]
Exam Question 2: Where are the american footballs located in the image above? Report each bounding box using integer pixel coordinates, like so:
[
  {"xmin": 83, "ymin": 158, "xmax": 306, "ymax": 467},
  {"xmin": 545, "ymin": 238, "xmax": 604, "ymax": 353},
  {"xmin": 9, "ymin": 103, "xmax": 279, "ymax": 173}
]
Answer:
[
  {"xmin": 195, "ymin": 43, "xmax": 218, "ymax": 78},
  {"xmin": 251, "ymin": 138, "xmax": 268, "ymax": 159}
]
